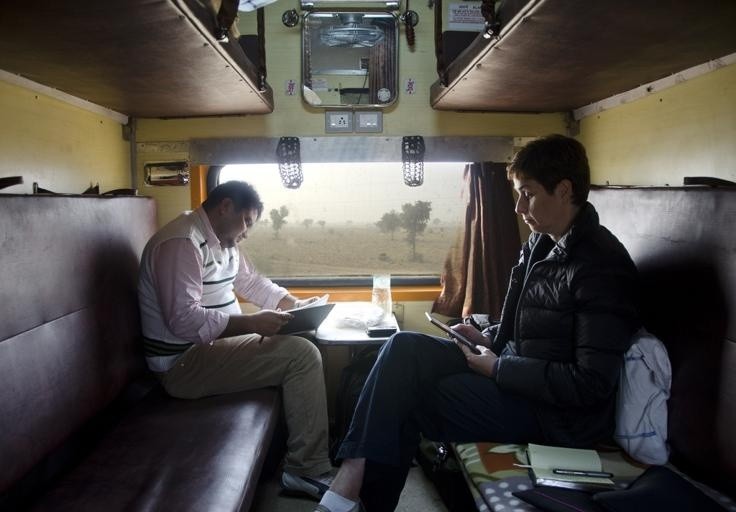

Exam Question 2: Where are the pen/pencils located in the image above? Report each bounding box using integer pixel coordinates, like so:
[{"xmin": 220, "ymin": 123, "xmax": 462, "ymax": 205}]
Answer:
[
  {"xmin": 258, "ymin": 307, "xmax": 281, "ymax": 344},
  {"xmin": 552, "ymin": 469, "xmax": 614, "ymax": 478}
]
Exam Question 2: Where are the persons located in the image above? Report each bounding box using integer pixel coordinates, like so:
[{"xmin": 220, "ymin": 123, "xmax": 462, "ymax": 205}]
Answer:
[
  {"xmin": 315, "ymin": 126, "xmax": 645, "ymax": 512},
  {"xmin": 133, "ymin": 180, "xmax": 343, "ymax": 500}
]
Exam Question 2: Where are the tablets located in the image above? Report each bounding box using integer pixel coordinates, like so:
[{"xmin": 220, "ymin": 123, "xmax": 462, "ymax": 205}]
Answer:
[{"xmin": 425, "ymin": 312, "xmax": 481, "ymax": 357}]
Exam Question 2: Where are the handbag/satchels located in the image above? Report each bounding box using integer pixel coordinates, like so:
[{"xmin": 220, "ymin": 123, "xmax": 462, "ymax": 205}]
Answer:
[{"xmin": 445, "ymin": 313, "xmax": 498, "ymax": 332}]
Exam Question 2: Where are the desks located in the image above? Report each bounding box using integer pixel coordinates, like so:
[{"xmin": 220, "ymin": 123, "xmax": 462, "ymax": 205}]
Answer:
[{"xmin": 315, "ymin": 298, "xmax": 401, "ymax": 347}]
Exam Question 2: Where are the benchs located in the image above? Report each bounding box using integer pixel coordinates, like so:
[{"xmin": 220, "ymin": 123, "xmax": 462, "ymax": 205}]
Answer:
[
  {"xmin": 1, "ymin": 192, "xmax": 282, "ymax": 512},
  {"xmin": 448, "ymin": 185, "xmax": 734, "ymax": 510}
]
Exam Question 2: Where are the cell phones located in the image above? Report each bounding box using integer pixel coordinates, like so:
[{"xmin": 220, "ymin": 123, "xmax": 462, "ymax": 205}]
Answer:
[{"xmin": 368, "ymin": 327, "xmax": 397, "ymax": 337}]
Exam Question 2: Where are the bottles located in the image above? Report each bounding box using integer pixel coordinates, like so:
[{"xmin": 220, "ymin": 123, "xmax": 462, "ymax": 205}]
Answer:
[{"xmin": 371, "ymin": 272, "xmax": 393, "ymax": 320}]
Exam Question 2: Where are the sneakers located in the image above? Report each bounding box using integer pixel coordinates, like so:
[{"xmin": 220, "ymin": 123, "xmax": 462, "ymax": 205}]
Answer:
[
  {"xmin": 314, "ymin": 501, "xmax": 359, "ymax": 511},
  {"xmin": 282, "ymin": 470, "xmax": 335, "ymax": 500}
]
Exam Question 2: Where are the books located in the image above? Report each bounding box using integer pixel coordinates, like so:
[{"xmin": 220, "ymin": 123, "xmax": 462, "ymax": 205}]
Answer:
[
  {"xmin": 523, "ymin": 444, "xmax": 618, "ymax": 495},
  {"xmin": 275, "ymin": 294, "xmax": 335, "ymax": 336}
]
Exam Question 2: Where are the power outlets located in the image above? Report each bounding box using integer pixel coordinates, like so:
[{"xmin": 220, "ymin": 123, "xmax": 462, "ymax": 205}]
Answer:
[{"xmin": 324, "ymin": 110, "xmax": 353, "ymax": 132}]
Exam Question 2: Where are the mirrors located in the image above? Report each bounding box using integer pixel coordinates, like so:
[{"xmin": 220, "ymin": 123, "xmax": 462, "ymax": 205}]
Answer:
[{"xmin": 297, "ymin": 7, "xmax": 403, "ymax": 110}]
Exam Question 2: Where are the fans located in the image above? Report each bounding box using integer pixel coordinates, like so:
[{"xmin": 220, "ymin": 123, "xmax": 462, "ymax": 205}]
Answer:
[{"xmin": 319, "ymin": 12, "xmax": 385, "ymax": 49}]
